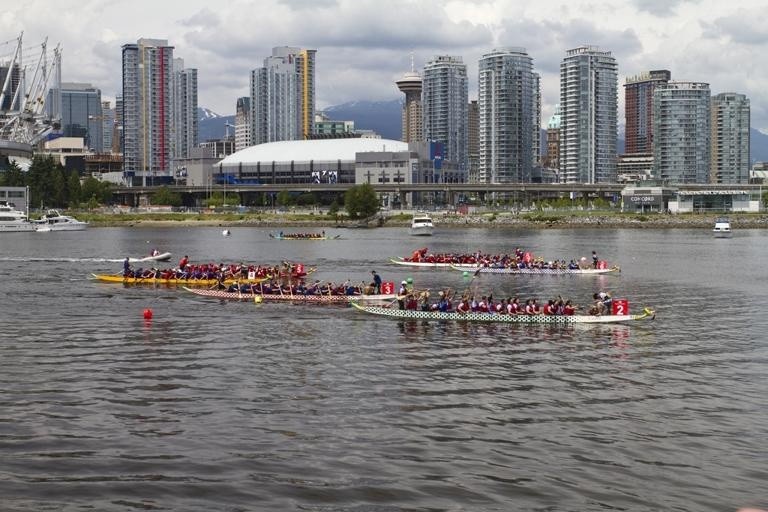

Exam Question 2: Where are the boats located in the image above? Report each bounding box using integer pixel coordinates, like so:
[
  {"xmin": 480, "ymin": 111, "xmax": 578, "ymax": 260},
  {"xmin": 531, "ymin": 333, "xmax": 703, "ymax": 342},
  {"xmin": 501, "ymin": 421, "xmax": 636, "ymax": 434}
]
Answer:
[
  {"xmin": 267, "ymin": 232, "xmax": 339, "ymax": 241},
  {"xmin": 178, "ymin": 282, "xmax": 430, "ymax": 303},
  {"xmin": 410, "ymin": 214, "xmax": 434, "ymax": 236},
  {"xmin": 712, "ymin": 222, "xmax": 731, "ymax": 238},
  {"xmin": 0, "ymin": 200, "xmax": 32, "ymax": 231},
  {"xmin": 391, "ymin": 255, "xmax": 617, "ymax": 275},
  {"xmin": 135, "ymin": 252, "xmax": 172, "ymax": 261},
  {"xmin": 90, "ymin": 264, "xmax": 318, "ymax": 285},
  {"xmin": 222, "ymin": 230, "xmax": 230, "ymax": 236},
  {"xmin": 34, "ymin": 210, "xmax": 89, "ymax": 231},
  {"xmin": 349, "ymin": 298, "xmax": 656, "ymax": 324}
]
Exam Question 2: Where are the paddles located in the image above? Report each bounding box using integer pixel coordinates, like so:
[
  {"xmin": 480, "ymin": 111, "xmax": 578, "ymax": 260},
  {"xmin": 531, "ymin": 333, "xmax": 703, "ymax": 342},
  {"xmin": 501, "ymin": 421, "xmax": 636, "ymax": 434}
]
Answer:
[{"xmin": 121, "ymin": 270, "xmax": 333, "ymax": 303}]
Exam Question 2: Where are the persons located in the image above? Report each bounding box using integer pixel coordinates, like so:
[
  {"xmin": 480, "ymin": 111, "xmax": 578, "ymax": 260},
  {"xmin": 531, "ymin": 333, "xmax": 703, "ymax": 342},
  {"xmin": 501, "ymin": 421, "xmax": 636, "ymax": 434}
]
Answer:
[
  {"xmin": 398, "ymin": 246, "xmax": 610, "ymax": 271},
  {"xmin": 121, "ymin": 248, "xmax": 381, "ymax": 308},
  {"xmin": 397, "ymin": 279, "xmax": 614, "ymax": 317},
  {"xmin": 278, "ymin": 230, "xmax": 327, "ymax": 238}
]
